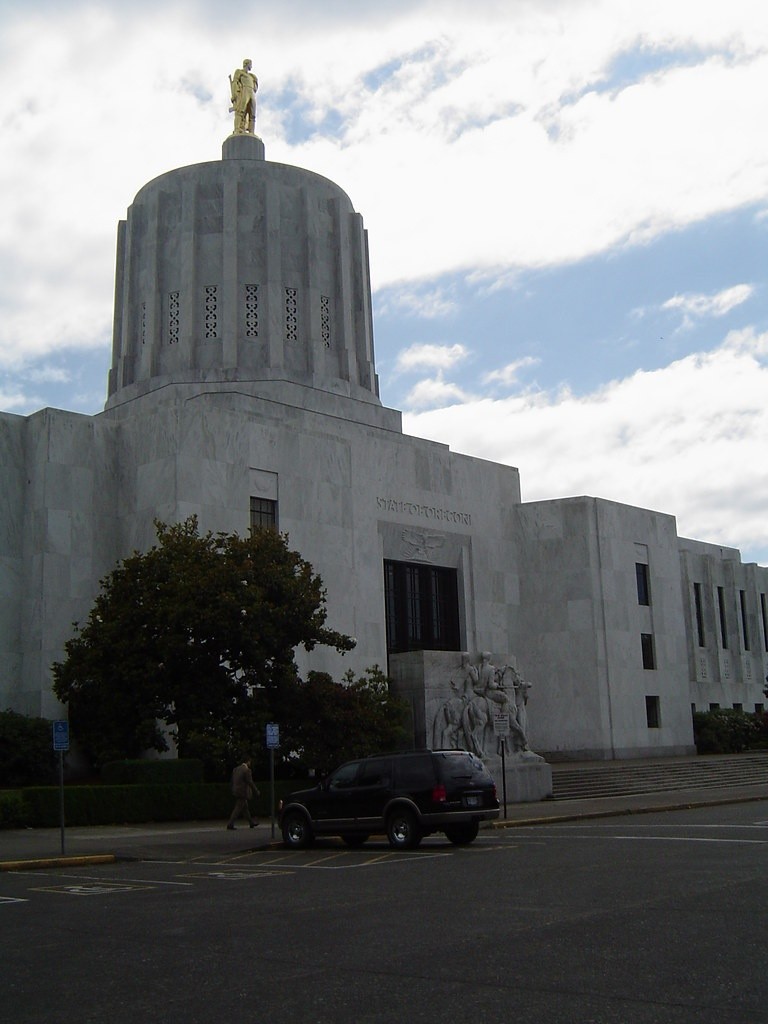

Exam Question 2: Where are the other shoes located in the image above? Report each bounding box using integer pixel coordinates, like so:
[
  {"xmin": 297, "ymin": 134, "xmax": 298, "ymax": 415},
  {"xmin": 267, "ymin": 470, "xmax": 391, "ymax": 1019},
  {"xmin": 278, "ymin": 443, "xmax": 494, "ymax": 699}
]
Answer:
[
  {"xmin": 250, "ymin": 822, "xmax": 259, "ymax": 828},
  {"xmin": 227, "ymin": 825, "xmax": 237, "ymax": 830}
]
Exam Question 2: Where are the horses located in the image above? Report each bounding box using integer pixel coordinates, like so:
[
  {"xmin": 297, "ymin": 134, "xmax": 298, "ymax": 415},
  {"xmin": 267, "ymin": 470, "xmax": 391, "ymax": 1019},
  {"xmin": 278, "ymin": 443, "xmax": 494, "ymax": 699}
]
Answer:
[{"xmin": 433, "ymin": 666, "xmax": 525, "ymax": 757}]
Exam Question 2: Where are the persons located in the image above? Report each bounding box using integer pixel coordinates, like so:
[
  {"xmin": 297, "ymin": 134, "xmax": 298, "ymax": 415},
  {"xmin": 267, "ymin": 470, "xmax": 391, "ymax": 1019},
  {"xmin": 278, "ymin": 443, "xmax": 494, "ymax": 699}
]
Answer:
[
  {"xmin": 452, "ymin": 652, "xmax": 512, "ymax": 714},
  {"xmin": 227, "ymin": 757, "xmax": 261, "ymax": 830},
  {"xmin": 231, "ymin": 60, "xmax": 258, "ymax": 136}
]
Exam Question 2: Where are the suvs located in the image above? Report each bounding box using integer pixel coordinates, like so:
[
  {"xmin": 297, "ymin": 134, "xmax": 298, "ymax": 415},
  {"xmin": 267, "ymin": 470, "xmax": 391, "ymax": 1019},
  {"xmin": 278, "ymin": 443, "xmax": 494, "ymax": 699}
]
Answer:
[{"xmin": 275, "ymin": 747, "xmax": 502, "ymax": 854}]
